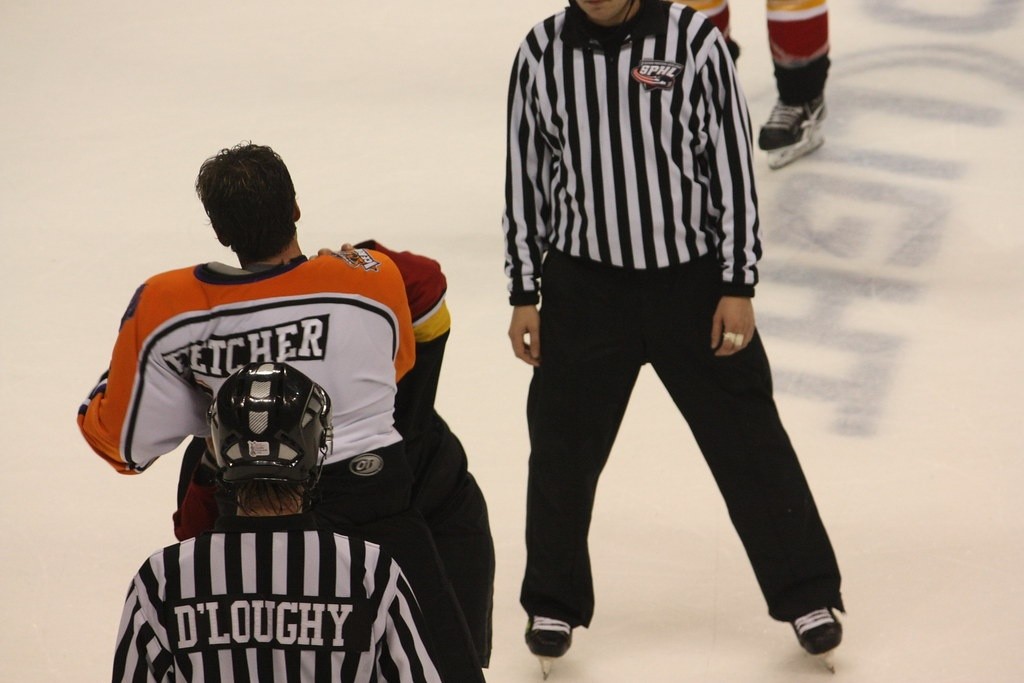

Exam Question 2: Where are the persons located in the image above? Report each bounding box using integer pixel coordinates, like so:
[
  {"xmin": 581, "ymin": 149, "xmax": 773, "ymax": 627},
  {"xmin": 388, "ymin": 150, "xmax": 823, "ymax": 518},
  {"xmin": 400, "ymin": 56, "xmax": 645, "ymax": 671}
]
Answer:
[
  {"xmin": 78, "ymin": 141, "xmax": 486, "ymax": 683},
  {"xmin": 112, "ymin": 360, "xmax": 449, "ymax": 683},
  {"xmin": 680, "ymin": 0, "xmax": 830, "ymax": 169},
  {"xmin": 503, "ymin": 0, "xmax": 848, "ymax": 677}
]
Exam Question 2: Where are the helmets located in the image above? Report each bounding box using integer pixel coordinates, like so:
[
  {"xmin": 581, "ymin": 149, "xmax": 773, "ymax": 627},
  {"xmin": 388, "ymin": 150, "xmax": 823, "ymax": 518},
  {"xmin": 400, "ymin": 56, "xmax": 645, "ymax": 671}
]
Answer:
[{"xmin": 205, "ymin": 361, "xmax": 334, "ymax": 491}]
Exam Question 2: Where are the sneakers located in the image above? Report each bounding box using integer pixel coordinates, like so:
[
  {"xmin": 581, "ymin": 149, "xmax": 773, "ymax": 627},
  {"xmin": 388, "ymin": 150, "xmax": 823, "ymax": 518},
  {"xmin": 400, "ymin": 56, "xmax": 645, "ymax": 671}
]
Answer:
[
  {"xmin": 726, "ymin": 38, "xmax": 740, "ymax": 71},
  {"xmin": 789, "ymin": 606, "xmax": 842, "ymax": 673},
  {"xmin": 525, "ymin": 613, "xmax": 573, "ymax": 681},
  {"xmin": 758, "ymin": 49, "xmax": 832, "ymax": 170}
]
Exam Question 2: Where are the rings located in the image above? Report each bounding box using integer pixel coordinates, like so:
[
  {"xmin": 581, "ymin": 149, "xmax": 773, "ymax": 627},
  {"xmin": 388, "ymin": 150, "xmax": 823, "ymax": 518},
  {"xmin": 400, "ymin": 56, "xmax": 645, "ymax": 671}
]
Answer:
[{"xmin": 723, "ymin": 332, "xmax": 744, "ymax": 346}]
